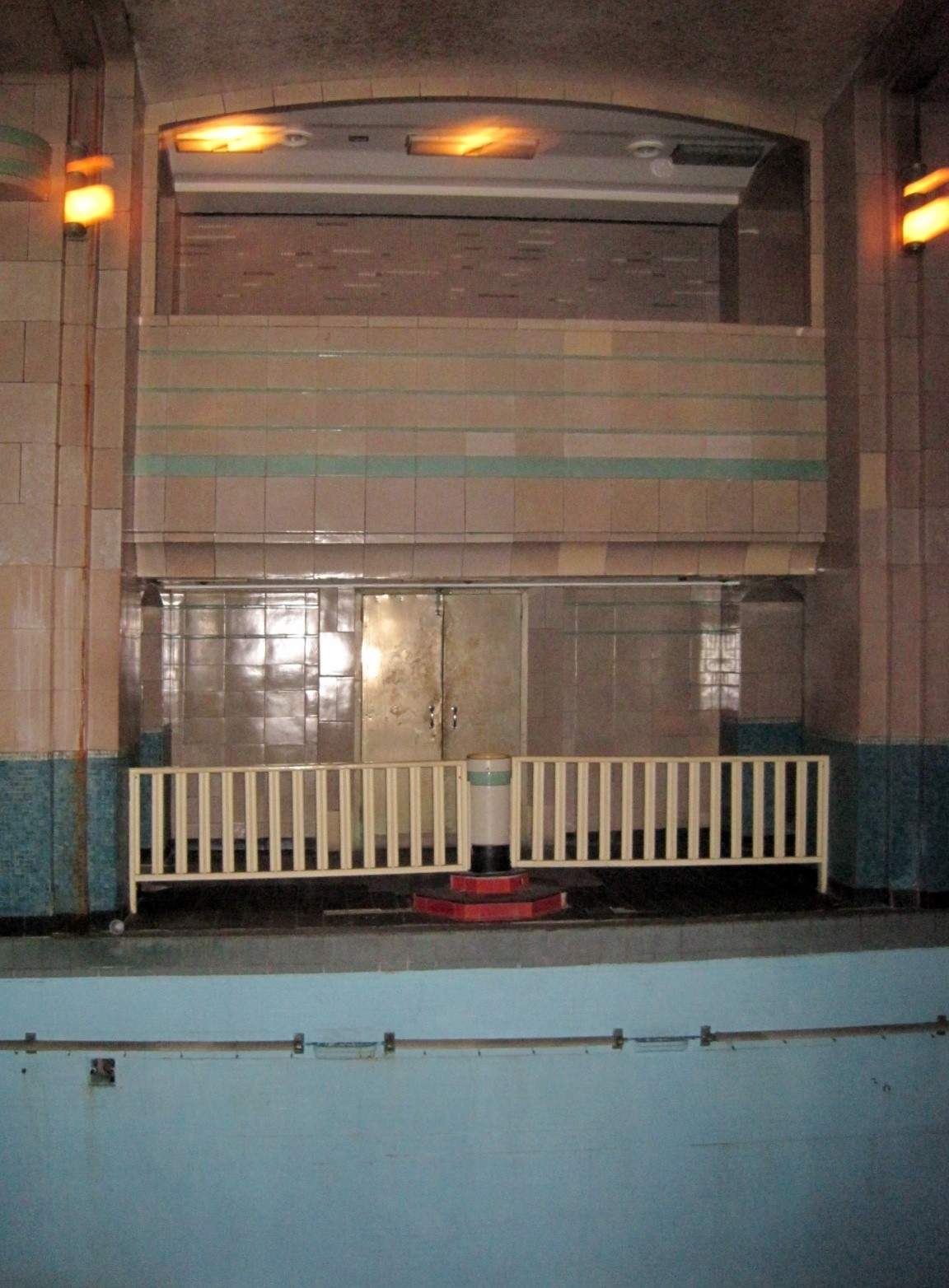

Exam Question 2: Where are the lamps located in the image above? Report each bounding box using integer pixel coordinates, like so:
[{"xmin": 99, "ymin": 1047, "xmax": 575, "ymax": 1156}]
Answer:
[
  {"xmin": 897, "ymin": 160, "xmax": 949, "ymax": 257},
  {"xmin": 58, "ymin": 141, "xmax": 116, "ymax": 243},
  {"xmin": 172, "ymin": 127, "xmax": 284, "ymax": 157},
  {"xmin": 404, "ymin": 130, "xmax": 540, "ymax": 162}
]
